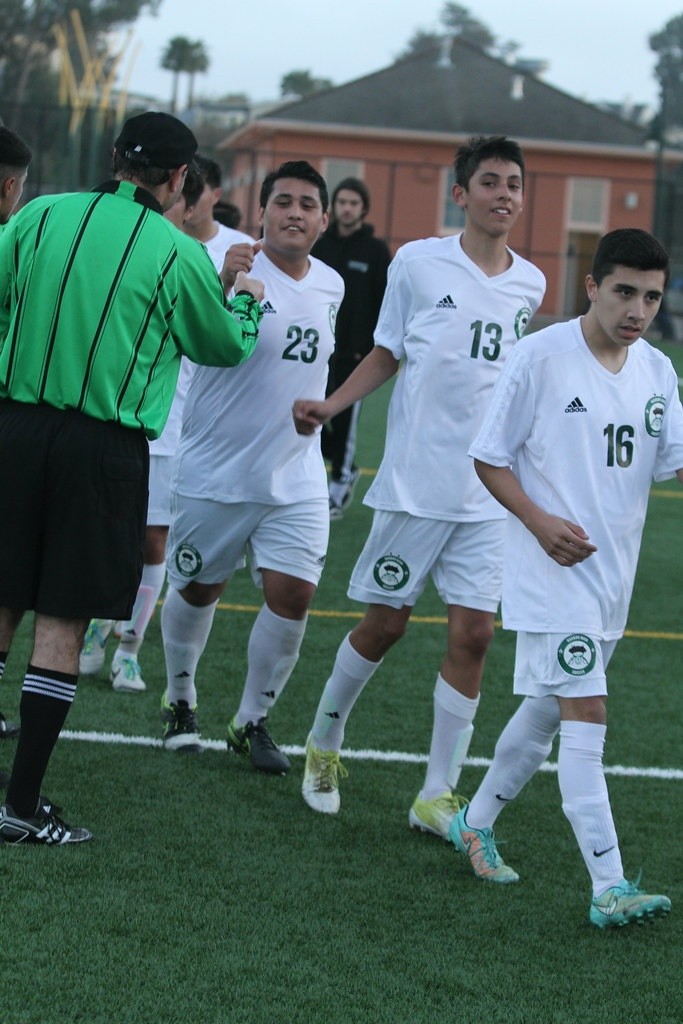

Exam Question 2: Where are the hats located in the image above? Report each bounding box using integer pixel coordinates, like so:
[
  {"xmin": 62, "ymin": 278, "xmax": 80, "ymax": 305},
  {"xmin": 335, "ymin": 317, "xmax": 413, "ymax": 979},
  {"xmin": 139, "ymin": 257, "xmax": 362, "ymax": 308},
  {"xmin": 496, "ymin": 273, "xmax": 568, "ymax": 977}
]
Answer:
[{"xmin": 114, "ymin": 111, "xmax": 199, "ymax": 170}]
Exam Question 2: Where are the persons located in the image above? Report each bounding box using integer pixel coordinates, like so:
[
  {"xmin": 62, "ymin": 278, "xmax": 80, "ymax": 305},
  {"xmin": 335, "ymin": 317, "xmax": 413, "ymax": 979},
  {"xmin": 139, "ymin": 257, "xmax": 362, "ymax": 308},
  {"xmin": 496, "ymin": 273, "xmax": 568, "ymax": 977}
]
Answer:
[
  {"xmin": 0, "ymin": 112, "xmax": 267, "ymax": 843},
  {"xmin": 78, "ymin": 152, "xmax": 394, "ymax": 773},
  {"xmin": 292, "ymin": 137, "xmax": 547, "ymax": 840},
  {"xmin": 448, "ymin": 229, "xmax": 683, "ymax": 928}
]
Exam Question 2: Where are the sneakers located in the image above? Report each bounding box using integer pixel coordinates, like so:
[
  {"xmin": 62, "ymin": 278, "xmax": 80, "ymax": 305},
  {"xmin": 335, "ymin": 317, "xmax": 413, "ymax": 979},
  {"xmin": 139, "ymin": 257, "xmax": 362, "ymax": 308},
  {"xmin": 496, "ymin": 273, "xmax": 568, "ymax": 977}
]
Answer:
[
  {"xmin": 0, "ymin": 712, "xmax": 21, "ymax": 736},
  {"xmin": 224, "ymin": 714, "xmax": 291, "ymax": 775},
  {"xmin": 448, "ymin": 804, "xmax": 519, "ymax": 884},
  {"xmin": 588, "ymin": 867, "xmax": 671, "ymax": 930},
  {"xmin": 34, "ymin": 796, "xmax": 63, "ymax": 818},
  {"xmin": 108, "ymin": 651, "xmax": 146, "ymax": 692},
  {"xmin": 0, "ymin": 795, "xmax": 92, "ymax": 846},
  {"xmin": 302, "ymin": 730, "xmax": 348, "ymax": 814},
  {"xmin": 408, "ymin": 788, "xmax": 470, "ymax": 840},
  {"xmin": 159, "ymin": 686, "xmax": 202, "ymax": 752},
  {"xmin": 78, "ymin": 616, "xmax": 116, "ymax": 673},
  {"xmin": 328, "ymin": 463, "xmax": 360, "ymax": 520}
]
